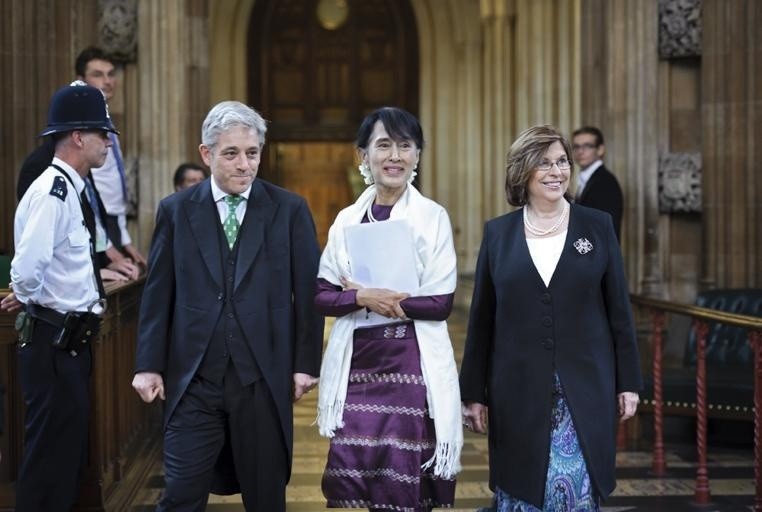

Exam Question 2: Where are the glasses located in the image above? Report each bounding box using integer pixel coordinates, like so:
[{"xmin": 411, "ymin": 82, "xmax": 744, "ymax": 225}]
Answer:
[{"xmin": 534, "ymin": 156, "xmax": 570, "ymax": 170}]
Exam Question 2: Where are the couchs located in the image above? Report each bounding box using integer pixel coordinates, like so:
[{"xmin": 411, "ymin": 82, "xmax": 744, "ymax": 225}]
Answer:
[{"xmin": 627, "ymin": 288, "xmax": 762, "ymax": 454}]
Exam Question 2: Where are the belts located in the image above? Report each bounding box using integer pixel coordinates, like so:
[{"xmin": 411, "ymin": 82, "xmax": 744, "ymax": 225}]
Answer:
[{"xmin": 23, "ymin": 303, "xmax": 64, "ymax": 329}]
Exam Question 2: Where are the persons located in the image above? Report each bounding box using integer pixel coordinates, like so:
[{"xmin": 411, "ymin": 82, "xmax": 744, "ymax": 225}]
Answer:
[
  {"xmin": 461, "ymin": 124, "xmax": 642, "ymax": 511},
  {"xmin": 171, "ymin": 161, "xmax": 206, "ymax": 192},
  {"xmin": 1, "ymin": 79, "xmax": 114, "ymax": 511},
  {"xmin": 313, "ymin": 107, "xmax": 457, "ymax": 512},
  {"xmin": 567, "ymin": 127, "xmax": 626, "ymax": 247},
  {"xmin": 16, "ymin": 81, "xmax": 141, "ymax": 284},
  {"xmin": 74, "ymin": 45, "xmax": 147, "ymax": 276},
  {"xmin": 130, "ymin": 101, "xmax": 324, "ymax": 512}
]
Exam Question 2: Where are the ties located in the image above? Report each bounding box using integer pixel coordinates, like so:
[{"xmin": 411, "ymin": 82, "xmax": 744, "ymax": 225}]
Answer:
[
  {"xmin": 109, "ymin": 117, "xmax": 128, "ymax": 202},
  {"xmin": 79, "ymin": 191, "xmax": 110, "ymax": 311},
  {"xmin": 221, "ymin": 194, "xmax": 245, "ymax": 250}
]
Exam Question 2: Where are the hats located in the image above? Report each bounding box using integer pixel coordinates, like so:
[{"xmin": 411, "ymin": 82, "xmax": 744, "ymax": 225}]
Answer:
[{"xmin": 36, "ymin": 79, "xmax": 120, "ymax": 137}]
